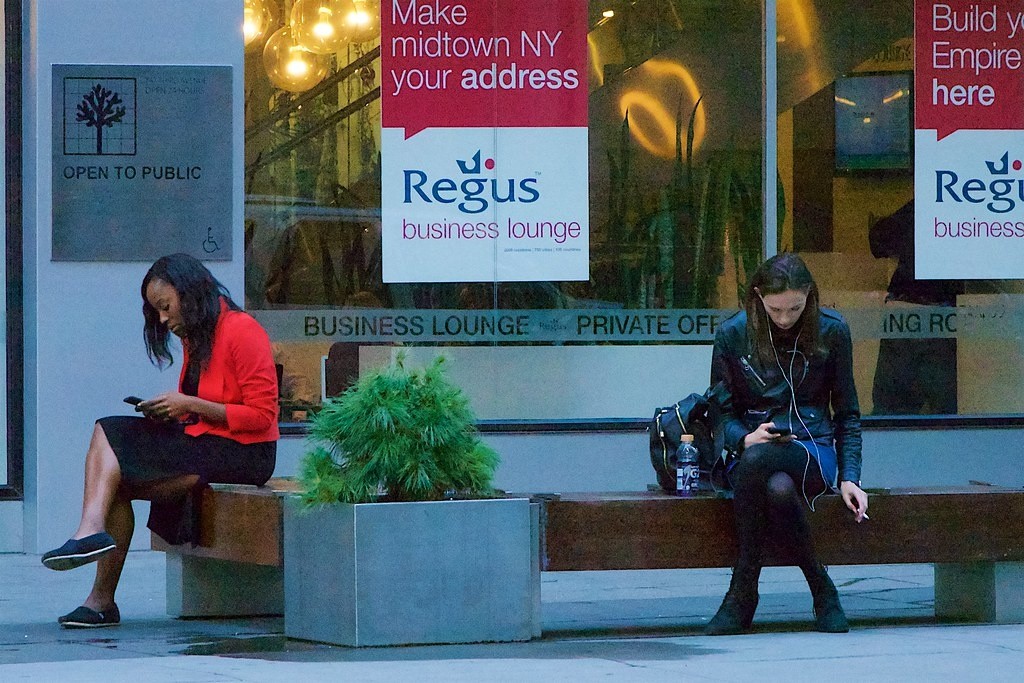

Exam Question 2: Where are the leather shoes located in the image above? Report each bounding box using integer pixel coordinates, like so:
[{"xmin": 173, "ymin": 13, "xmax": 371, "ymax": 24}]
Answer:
[
  {"xmin": 704, "ymin": 549, "xmax": 766, "ymax": 634},
  {"xmin": 800, "ymin": 558, "xmax": 849, "ymax": 633}
]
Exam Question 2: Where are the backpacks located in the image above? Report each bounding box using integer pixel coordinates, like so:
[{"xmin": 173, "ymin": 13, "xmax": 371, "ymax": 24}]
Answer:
[{"xmin": 650, "ymin": 380, "xmax": 726, "ymax": 494}]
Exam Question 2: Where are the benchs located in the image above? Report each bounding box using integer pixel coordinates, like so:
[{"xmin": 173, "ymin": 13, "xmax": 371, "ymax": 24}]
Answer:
[
  {"xmin": 149, "ymin": 475, "xmax": 313, "ymax": 620},
  {"xmin": 507, "ymin": 480, "xmax": 1024, "ymax": 622}
]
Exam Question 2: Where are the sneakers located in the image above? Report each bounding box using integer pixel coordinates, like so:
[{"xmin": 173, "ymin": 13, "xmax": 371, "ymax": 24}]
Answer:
[
  {"xmin": 57, "ymin": 601, "xmax": 122, "ymax": 631},
  {"xmin": 40, "ymin": 531, "xmax": 117, "ymax": 572}
]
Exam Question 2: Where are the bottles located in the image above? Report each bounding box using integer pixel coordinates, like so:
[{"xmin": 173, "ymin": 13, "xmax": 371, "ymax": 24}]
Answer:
[{"xmin": 676, "ymin": 435, "xmax": 699, "ymax": 495}]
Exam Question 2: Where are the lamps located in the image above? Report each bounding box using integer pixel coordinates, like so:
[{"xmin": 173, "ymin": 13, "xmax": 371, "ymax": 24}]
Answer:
[{"xmin": 244, "ymin": 0, "xmax": 380, "ymax": 92}]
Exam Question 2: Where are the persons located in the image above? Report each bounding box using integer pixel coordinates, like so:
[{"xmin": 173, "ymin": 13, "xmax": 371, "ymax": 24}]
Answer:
[
  {"xmin": 868, "ymin": 196, "xmax": 959, "ymax": 415},
  {"xmin": 41, "ymin": 254, "xmax": 282, "ymax": 628},
  {"xmin": 706, "ymin": 250, "xmax": 869, "ymax": 635}
]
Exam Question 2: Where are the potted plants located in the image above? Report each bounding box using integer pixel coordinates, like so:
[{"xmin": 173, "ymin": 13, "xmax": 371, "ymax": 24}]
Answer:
[{"xmin": 284, "ymin": 341, "xmax": 538, "ymax": 649}]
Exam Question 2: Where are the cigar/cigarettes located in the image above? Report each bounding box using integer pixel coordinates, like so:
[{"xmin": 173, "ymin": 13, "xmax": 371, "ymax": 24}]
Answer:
[{"xmin": 857, "ymin": 508, "xmax": 870, "ymax": 520}]
